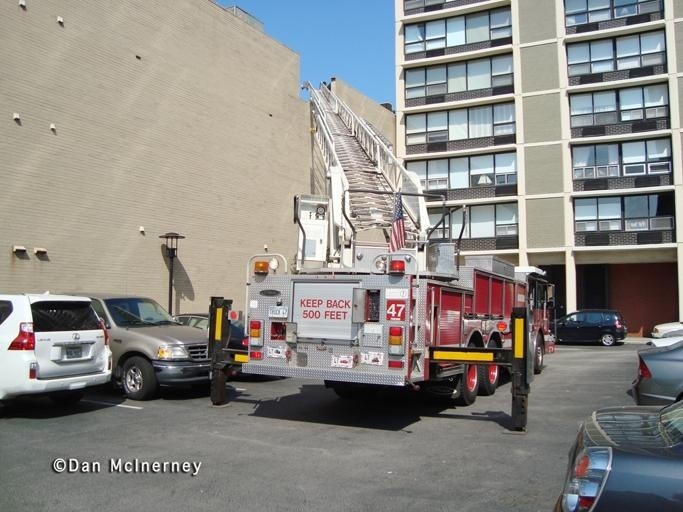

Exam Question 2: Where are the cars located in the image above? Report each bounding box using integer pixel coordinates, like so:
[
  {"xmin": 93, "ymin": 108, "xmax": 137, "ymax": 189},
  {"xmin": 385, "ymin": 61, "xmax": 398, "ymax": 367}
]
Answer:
[
  {"xmin": 0, "ymin": 291, "xmax": 281, "ymax": 411},
  {"xmin": 556, "ymin": 322, "xmax": 683, "ymax": 512}
]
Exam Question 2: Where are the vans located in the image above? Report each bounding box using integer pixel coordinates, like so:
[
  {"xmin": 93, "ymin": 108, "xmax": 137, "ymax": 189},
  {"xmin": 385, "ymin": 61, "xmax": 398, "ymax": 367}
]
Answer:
[{"xmin": 549, "ymin": 308, "xmax": 628, "ymax": 345}]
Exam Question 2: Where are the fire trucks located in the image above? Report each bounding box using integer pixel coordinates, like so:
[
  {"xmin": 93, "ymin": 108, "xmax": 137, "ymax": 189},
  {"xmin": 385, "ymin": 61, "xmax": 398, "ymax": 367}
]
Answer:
[{"xmin": 207, "ymin": 81, "xmax": 555, "ymax": 430}]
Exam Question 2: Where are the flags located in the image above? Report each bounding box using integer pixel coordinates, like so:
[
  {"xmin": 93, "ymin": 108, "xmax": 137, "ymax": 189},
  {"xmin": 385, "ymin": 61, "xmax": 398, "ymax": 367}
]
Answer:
[{"xmin": 387, "ymin": 190, "xmax": 407, "ymax": 255}]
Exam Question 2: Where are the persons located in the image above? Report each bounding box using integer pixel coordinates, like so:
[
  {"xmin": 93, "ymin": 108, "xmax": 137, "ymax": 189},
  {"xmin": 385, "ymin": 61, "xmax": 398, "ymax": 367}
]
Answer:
[{"xmin": 536, "ymin": 295, "xmax": 565, "ymax": 319}]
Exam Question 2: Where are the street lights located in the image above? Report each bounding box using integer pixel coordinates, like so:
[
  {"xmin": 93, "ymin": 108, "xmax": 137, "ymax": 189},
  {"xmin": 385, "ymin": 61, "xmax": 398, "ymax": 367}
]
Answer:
[{"xmin": 159, "ymin": 232, "xmax": 185, "ymax": 315}]
